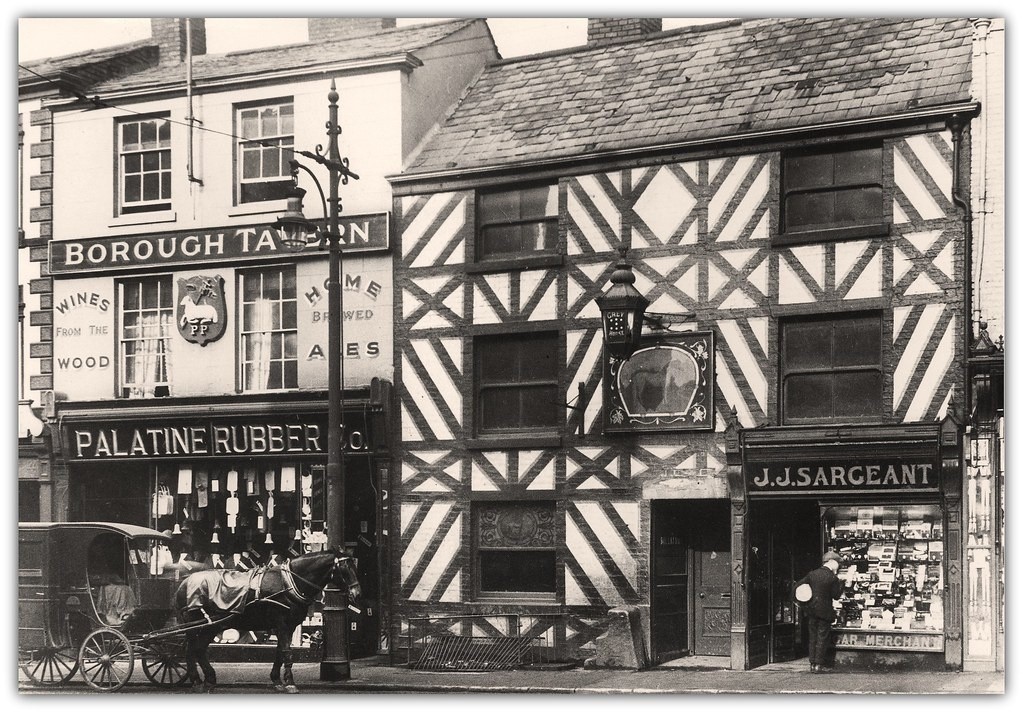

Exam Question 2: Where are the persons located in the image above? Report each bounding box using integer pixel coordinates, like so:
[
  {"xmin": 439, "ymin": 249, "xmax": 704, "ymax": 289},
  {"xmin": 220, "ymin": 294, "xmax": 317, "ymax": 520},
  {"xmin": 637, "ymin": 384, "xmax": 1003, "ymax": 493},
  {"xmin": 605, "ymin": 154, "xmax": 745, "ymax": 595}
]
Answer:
[
  {"xmin": 790, "ymin": 551, "xmax": 846, "ymax": 674},
  {"xmin": 89, "ymin": 543, "xmax": 135, "ymax": 626}
]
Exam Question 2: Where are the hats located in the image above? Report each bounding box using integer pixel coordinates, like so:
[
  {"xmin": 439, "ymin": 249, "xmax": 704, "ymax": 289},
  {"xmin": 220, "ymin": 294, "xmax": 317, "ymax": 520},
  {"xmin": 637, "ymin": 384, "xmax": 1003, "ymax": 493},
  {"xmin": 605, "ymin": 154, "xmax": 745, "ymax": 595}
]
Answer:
[{"xmin": 822, "ymin": 551, "xmax": 840, "ymax": 563}]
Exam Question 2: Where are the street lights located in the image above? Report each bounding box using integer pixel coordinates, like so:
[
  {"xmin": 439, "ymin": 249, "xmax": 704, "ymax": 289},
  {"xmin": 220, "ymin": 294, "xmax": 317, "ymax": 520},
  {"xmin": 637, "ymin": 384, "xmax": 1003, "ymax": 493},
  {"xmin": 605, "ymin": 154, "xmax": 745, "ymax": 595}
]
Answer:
[{"xmin": 273, "ymin": 77, "xmax": 361, "ymax": 683}]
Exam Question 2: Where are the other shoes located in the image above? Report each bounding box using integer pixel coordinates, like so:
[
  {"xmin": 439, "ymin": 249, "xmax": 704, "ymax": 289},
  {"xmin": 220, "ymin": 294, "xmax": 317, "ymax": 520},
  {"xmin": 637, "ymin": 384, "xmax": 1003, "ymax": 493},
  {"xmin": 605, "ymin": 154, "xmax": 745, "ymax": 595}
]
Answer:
[
  {"xmin": 814, "ymin": 664, "xmax": 829, "ymax": 673},
  {"xmin": 810, "ymin": 663, "xmax": 816, "ymax": 672},
  {"xmin": 109, "ymin": 617, "xmax": 125, "ymax": 626}
]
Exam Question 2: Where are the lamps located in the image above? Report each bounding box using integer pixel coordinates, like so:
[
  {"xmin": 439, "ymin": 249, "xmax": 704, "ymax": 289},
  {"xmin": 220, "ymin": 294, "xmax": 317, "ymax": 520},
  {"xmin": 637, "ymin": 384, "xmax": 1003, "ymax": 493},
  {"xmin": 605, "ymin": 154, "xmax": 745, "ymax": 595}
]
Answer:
[{"xmin": 595, "ymin": 247, "xmax": 668, "ymax": 363}]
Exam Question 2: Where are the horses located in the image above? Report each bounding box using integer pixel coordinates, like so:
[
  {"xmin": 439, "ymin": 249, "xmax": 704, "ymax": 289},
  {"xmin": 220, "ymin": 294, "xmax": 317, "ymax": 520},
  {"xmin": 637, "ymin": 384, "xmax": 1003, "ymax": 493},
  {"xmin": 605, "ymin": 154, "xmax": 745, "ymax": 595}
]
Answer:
[{"xmin": 175, "ymin": 544, "xmax": 361, "ymax": 692}]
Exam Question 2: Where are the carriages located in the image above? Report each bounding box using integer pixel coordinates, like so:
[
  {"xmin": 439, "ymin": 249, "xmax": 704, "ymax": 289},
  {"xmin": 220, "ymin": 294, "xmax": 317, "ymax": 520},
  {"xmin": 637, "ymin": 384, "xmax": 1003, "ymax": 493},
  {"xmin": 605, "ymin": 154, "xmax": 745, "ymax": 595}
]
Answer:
[{"xmin": 18, "ymin": 522, "xmax": 363, "ymax": 692}]
